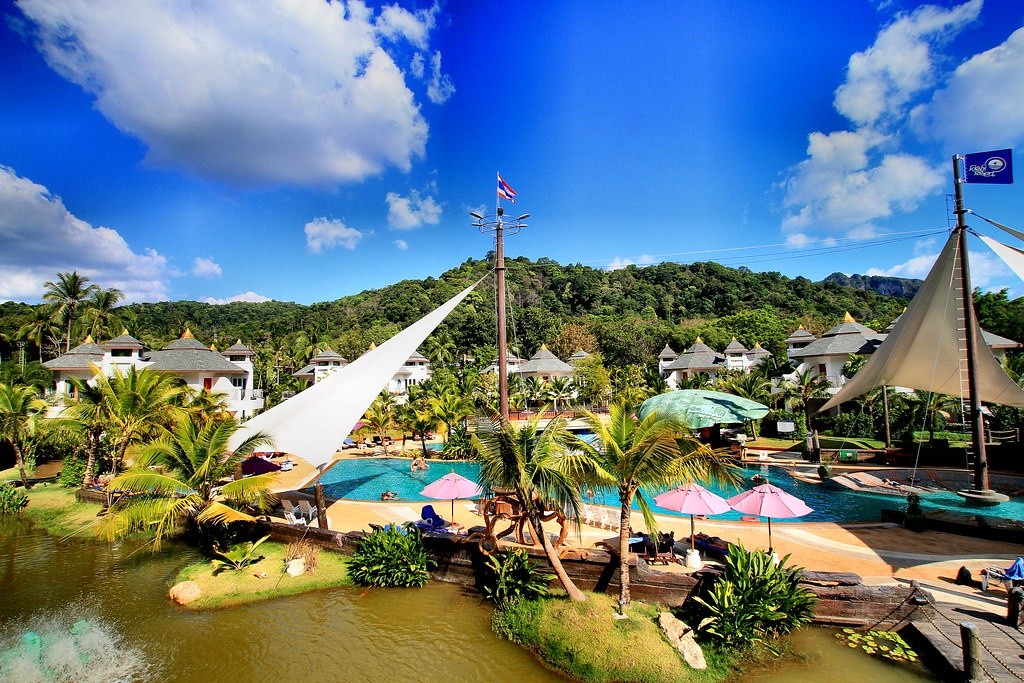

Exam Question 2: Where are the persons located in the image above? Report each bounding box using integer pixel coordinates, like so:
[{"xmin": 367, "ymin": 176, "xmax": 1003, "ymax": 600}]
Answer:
[
  {"xmin": 750, "ymin": 473, "xmax": 769, "ymax": 488},
  {"xmin": 382, "ymin": 491, "xmax": 398, "ymax": 500},
  {"xmin": 690, "ymin": 532, "xmax": 732, "ymax": 550},
  {"xmin": 94, "ymin": 478, "xmax": 109, "ymax": 486},
  {"xmin": 881, "ymin": 478, "xmax": 909, "ymax": 492},
  {"xmin": 411, "ymin": 456, "xmax": 427, "ymax": 472}
]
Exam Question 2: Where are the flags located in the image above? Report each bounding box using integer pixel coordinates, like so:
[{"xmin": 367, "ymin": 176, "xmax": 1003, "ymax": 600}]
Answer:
[{"xmin": 496, "ymin": 174, "xmax": 517, "ymax": 203}]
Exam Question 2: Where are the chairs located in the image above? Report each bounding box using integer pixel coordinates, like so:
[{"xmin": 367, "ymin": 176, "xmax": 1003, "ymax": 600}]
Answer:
[
  {"xmin": 601, "ymin": 531, "xmax": 750, "ymax": 559},
  {"xmin": 368, "ymin": 523, "xmax": 384, "ymax": 534},
  {"xmin": 979, "ymin": 556, "xmax": 1023, "ymax": 592},
  {"xmin": 406, "ymin": 505, "xmax": 448, "ymax": 533},
  {"xmin": 342, "ymin": 435, "xmax": 394, "ymax": 448},
  {"xmin": 298, "ymin": 500, "xmax": 318, "ymax": 520},
  {"xmin": 281, "ymin": 498, "xmax": 300, "ymax": 518}
]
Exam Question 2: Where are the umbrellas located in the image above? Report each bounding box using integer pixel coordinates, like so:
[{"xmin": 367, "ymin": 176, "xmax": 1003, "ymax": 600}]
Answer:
[
  {"xmin": 726, "ymin": 480, "xmax": 814, "ymax": 548},
  {"xmin": 653, "ymin": 480, "xmax": 732, "ymax": 552},
  {"xmin": 419, "ymin": 473, "xmax": 484, "ymax": 529}
]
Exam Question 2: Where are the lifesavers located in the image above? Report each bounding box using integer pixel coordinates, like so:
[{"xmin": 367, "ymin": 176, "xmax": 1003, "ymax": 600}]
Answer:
[{"xmin": 740, "ymin": 516, "xmax": 761, "ymax": 522}]
